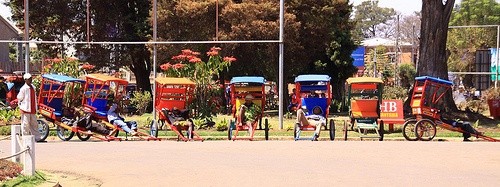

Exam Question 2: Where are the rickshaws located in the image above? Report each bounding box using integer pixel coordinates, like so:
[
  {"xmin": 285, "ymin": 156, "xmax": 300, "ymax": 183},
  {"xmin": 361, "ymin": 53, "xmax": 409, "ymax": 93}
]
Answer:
[
  {"xmin": 150, "ymin": 77, "xmax": 205, "ymax": 142},
  {"xmin": 293, "ymin": 74, "xmax": 336, "ymax": 141},
  {"xmin": 228, "ymin": 76, "xmax": 269, "ymax": 140},
  {"xmin": 34, "ymin": 72, "xmax": 162, "ymax": 142},
  {"xmin": 342, "ymin": 76, "xmax": 384, "ymax": 141},
  {"xmin": 401, "ymin": 75, "xmax": 500, "ymax": 142}
]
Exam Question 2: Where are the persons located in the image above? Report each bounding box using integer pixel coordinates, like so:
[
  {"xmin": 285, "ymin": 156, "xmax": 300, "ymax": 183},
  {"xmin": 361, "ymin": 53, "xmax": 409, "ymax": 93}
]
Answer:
[
  {"xmin": 443, "ymin": 117, "xmax": 485, "ymax": 141},
  {"xmin": 309, "ymin": 91, "xmax": 319, "ymax": 98},
  {"xmin": 68, "ymin": 113, "xmax": 116, "ymax": 136},
  {"xmin": 0, "ymin": 77, "xmax": 8, "ymax": 104},
  {"xmin": 108, "ymin": 102, "xmax": 137, "ymax": 135},
  {"xmin": 168, "ymin": 107, "xmax": 192, "ymax": 139},
  {"xmin": 117, "ymin": 90, "xmax": 134, "ymax": 100},
  {"xmin": 238, "ymin": 94, "xmax": 262, "ymax": 141},
  {"xmin": 296, "ymin": 106, "xmax": 327, "ymax": 141},
  {"xmin": 288, "ymin": 89, "xmax": 298, "ymax": 112},
  {"xmin": 17, "ymin": 73, "xmax": 47, "ymax": 143}
]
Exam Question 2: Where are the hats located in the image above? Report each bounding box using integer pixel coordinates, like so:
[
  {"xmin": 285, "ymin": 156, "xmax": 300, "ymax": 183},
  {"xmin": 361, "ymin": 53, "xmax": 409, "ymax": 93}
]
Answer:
[
  {"xmin": 246, "ymin": 94, "xmax": 253, "ymax": 99},
  {"xmin": 23, "ymin": 73, "xmax": 32, "ymax": 80}
]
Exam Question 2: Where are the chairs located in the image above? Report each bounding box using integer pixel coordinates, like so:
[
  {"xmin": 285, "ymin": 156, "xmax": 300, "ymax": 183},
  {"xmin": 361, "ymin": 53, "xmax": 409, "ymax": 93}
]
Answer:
[
  {"xmin": 87, "ymin": 97, "xmax": 109, "ymax": 118},
  {"xmin": 301, "ymin": 98, "xmax": 327, "ymax": 118},
  {"xmin": 351, "ymin": 99, "xmax": 378, "ymax": 119},
  {"xmin": 43, "ymin": 97, "xmax": 65, "ymax": 117},
  {"xmin": 157, "ymin": 99, "xmax": 185, "ymax": 119},
  {"xmin": 236, "ymin": 99, "xmax": 262, "ymax": 119}
]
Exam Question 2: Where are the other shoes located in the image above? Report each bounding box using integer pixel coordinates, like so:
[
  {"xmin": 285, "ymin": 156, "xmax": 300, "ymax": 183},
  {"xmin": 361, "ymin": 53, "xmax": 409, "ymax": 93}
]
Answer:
[
  {"xmin": 295, "ymin": 136, "xmax": 299, "ymax": 140},
  {"xmin": 238, "ymin": 122, "xmax": 242, "ymax": 127},
  {"xmin": 249, "ymin": 137, "xmax": 254, "ymax": 141},
  {"xmin": 312, "ymin": 137, "xmax": 317, "ymax": 141},
  {"xmin": 36, "ymin": 139, "xmax": 47, "ymax": 142},
  {"xmin": 463, "ymin": 138, "xmax": 470, "ymax": 141},
  {"xmin": 130, "ymin": 130, "xmax": 137, "ymax": 135}
]
